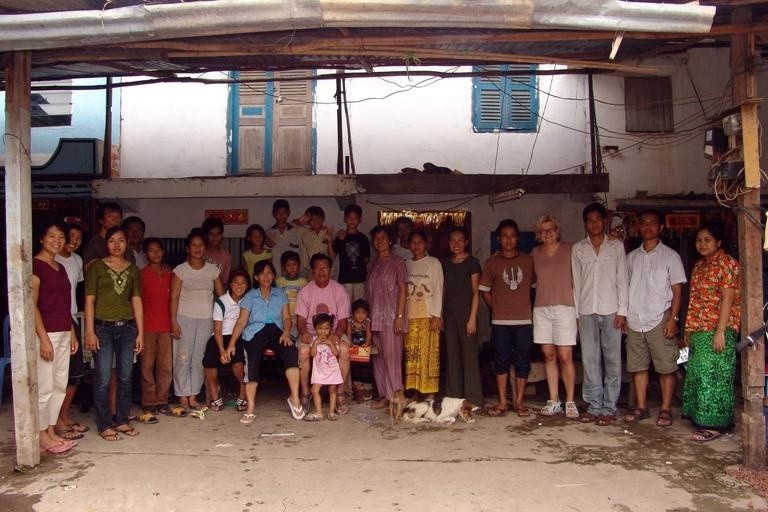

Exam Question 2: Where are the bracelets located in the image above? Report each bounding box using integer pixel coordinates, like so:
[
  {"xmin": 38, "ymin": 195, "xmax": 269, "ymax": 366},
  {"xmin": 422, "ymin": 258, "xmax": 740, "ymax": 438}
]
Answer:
[{"xmin": 715, "ymin": 326, "xmax": 726, "ymax": 332}]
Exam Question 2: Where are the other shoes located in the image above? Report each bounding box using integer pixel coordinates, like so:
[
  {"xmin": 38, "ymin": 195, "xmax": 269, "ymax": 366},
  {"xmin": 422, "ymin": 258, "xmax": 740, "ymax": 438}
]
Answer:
[{"xmin": 369, "ymin": 400, "xmax": 389, "ymax": 409}]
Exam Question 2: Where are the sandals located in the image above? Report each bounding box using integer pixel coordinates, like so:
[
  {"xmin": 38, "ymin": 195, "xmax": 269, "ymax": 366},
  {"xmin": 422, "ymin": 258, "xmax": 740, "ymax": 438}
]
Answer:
[
  {"xmin": 596, "ymin": 414, "xmax": 617, "ymax": 425},
  {"xmin": 541, "ymin": 400, "xmax": 562, "ymax": 416},
  {"xmin": 565, "ymin": 401, "xmax": 580, "ymax": 418},
  {"xmin": 655, "ymin": 409, "xmax": 672, "ymax": 427},
  {"xmin": 576, "ymin": 413, "xmax": 598, "ymax": 423},
  {"xmin": 623, "ymin": 408, "xmax": 649, "ymax": 423}
]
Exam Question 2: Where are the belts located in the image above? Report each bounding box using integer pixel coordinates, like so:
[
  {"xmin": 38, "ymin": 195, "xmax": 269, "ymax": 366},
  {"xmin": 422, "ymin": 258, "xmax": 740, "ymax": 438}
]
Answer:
[{"xmin": 93, "ymin": 317, "xmax": 135, "ymax": 328}]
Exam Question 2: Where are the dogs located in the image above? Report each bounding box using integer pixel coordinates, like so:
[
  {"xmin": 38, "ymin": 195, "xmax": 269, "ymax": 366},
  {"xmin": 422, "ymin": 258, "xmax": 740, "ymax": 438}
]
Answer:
[{"xmin": 401, "ymin": 396, "xmax": 482, "ymax": 426}]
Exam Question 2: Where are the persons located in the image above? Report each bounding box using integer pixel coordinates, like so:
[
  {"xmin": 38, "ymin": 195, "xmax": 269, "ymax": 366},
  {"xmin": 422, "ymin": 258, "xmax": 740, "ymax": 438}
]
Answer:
[
  {"xmin": 56, "ymin": 197, "xmax": 689, "ymax": 444},
  {"xmin": 679, "ymin": 220, "xmax": 742, "ymax": 442},
  {"xmin": 32, "ymin": 218, "xmax": 81, "ymax": 456}
]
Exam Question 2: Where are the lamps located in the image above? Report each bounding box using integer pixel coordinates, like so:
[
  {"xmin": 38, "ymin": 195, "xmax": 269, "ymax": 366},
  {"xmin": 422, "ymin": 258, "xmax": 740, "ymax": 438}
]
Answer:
[{"xmin": 488, "ymin": 189, "xmax": 525, "ymax": 205}]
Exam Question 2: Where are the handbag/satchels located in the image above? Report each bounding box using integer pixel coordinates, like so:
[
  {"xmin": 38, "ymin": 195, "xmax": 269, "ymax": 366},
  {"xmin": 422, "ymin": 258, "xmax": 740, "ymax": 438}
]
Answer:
[{"xmin": 479, "ymin": 341, "xmax": 503, "ymax": 377}]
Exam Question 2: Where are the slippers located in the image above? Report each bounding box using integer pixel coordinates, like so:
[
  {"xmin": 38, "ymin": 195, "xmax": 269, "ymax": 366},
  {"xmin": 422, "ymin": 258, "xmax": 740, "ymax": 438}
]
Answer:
[
  {"xmin": 488, "ymin": 403, "xmax": 511, "ymax": 417},
  {"xmin": 41, "ymin": 439, "xmax": 79, "ymax": 452},
  {"xmin": 693, "ymin": 428, "xmax": 721, "ymax": 441},
  {"xmin": 239, "ymin": 413, "xmax": 257, "ymax": 424},
  {"xmin": 236, "ymin": 397, "xmax": 249, "ymax": 411},
  {"xmin": 304, "ymin": 413, "xmax": 322, "ymax": 420},
  {"xmin": 135, "ymin": 409, "xmax": 159, "ymax": 424},
  {"xmin": 55, "ymin": 429, "xmax": 83, "ymax": 440},
  {"xmin": 99, "ymin": 431, "xmax": 124, "ymax": 441},
  {"xmin": 517, "ymin": 407, "xmax": 530, "ymax": 418},
  {"xmin": 113, "ymin": 426, "xmax": 140, "ymax": 437},
  {"xmin": 336, "ymin": 395, "xmax": 350, "ymax": 414},
  {"xmin": 209, "ymin": 398, "xmax": 226, "ymax": 411},
  {"xmin": 299, "ymin": 392, "xmax": 313, "ymax": 413},
  {"xmin": 286, "ymin": 397, "xmax": 305, "ymax": 420},
  {"xmin": 361, "ymin": 392, "xmax": 373, "ymax": 400},
  {"xmin": 188, "ymin": 409, "xmax": 205, "ymax": 418},
  {"xmin": 68, "ymin": 422, "xmax": 89, "ymax": 432},
  {"xmin": 189, "ymin": 403, "xmax": 209, "ymax": 412},
  {"xmin": 170, "ymin": 404, "xmax": 187, "ymax": 416},
  {"xmin": 328, "ymin": 412, "xmax": 337, "ymax": 420}
]
